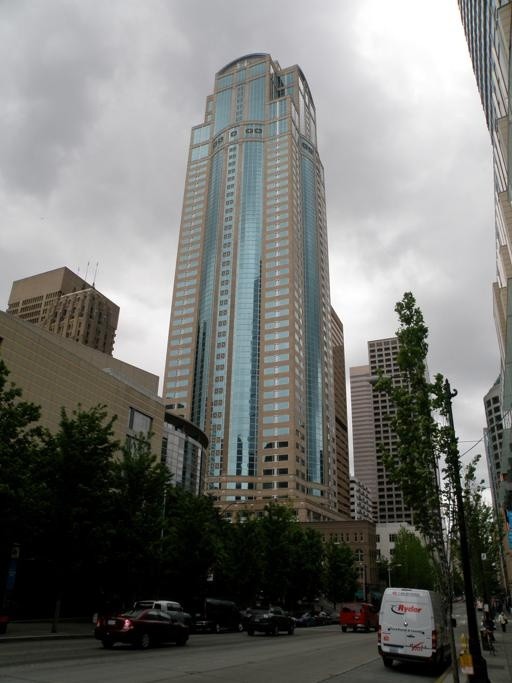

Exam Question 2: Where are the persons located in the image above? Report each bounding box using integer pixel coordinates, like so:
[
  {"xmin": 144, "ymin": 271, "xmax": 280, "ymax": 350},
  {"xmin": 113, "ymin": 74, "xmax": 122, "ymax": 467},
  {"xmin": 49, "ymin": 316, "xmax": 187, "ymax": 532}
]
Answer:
[{"xmin": 499, "ymin": 612, "xmax": 507, "ymax": 632}]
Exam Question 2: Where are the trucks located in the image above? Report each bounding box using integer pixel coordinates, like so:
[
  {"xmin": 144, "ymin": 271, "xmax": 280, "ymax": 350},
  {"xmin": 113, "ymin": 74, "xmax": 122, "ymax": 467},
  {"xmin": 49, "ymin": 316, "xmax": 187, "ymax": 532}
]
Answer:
[{"xmin": 378, "ymin": 587, "xmax": 454, "ymax": 672}]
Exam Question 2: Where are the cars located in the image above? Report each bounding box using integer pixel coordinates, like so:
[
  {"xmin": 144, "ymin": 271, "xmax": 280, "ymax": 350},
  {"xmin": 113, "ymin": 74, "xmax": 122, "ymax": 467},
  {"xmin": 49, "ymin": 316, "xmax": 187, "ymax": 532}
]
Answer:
[
  {"xmin": 341, "ymin": 601, "xmax": 378, "ymax": 634},
  {"xmin": 88, "ymin": 599, "xmax": 302, "ymax": 646}
]
